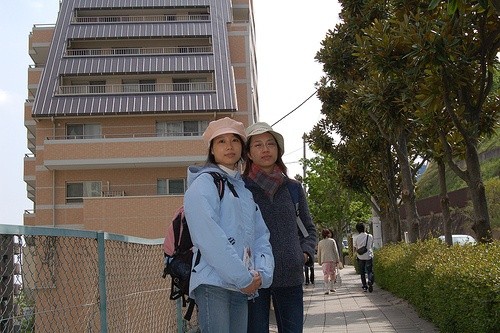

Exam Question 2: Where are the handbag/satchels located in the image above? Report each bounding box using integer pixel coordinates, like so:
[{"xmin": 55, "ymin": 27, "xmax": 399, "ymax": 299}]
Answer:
[
  {"xmin": 357, "ymin": 246, "xmax": 367, "ymax": 255},
  {"xmin": 335, "ymin": 263, "xmax": 343, "ymax": 288}
]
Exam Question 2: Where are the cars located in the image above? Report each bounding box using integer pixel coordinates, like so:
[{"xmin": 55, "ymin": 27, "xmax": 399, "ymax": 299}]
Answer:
[{"xmin": 438, "ymin": 235, "xmax": 478, "ymax": 248}]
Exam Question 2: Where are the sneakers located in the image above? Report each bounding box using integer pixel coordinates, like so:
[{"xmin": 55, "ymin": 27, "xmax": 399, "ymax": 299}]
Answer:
[{"xmin": 368, "ymin": 281, "xmax": 373, "ymax": 292}]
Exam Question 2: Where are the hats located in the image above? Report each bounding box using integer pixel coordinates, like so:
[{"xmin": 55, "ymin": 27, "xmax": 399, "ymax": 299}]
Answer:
[
  {"xmin": 201, "ymin": 117, "xmax": 248, "ymax": 147},
  {"xmin": 243, "ymin": 121, "xmax": 285, "ymax": 157}
]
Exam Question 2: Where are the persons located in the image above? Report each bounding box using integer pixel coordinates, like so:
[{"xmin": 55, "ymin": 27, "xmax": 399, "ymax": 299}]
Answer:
[
  {"xmin": 318, "ymin": 229, "xmax": 340, "ymax": 294},
  {"xmin": 241, "ymin": 122, "xmax": 316, "ymax": 333},
  {"xmin": 353, "ymin": 223, "xmax": 374, "ymax": 293},
  {"xmin": 304, "ymin": 262, "xmax": 315, "ymax": 285},
  {"xmin": 183, "ymin": 117, "xmax": 274, "ymax": 333}
]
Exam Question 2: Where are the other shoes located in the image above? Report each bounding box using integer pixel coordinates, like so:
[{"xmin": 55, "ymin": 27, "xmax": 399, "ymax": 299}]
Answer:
[{"xmin": 363, "ymin": 288, "xmax": 367, "ymax": 292}]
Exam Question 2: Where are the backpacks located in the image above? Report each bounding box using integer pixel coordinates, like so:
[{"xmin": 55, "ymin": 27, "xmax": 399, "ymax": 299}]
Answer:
[{"xmin": 162, "ymin": 170, "xmax": 225, "ymax": 296}]
